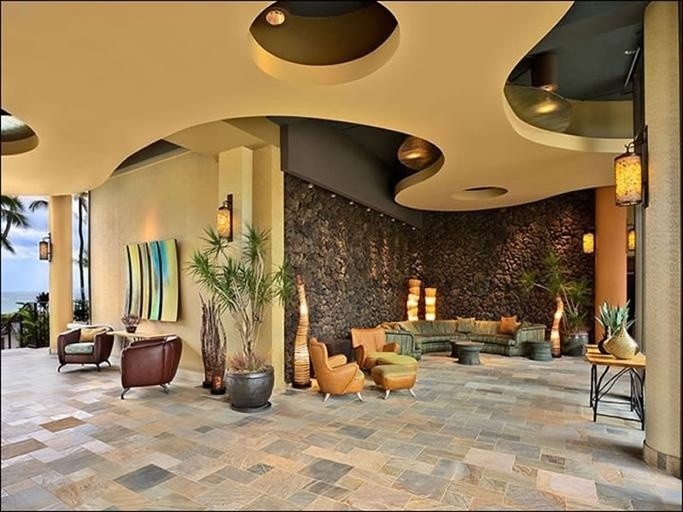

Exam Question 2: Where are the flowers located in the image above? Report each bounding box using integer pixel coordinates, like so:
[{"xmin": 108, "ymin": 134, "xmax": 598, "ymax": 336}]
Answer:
[{"xmin": 121, "ymin": 313, "xmax": 139, "ymax": 326}]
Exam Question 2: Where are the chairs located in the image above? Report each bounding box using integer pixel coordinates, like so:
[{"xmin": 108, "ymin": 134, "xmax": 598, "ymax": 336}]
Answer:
[
  {"xmin": 56, "ymin": 326, "xmax": 114, "ymax": 371},
  {"xmin": 120, "ymin": 334, "xmax": 182, "ymax": 399},
  {"xmin": 350, "ymin": 327, "xmax": 401, "ymax": 375},
  {"xmin": 308, "ymin": 336, "xmax": 365, "ymax": 402}
]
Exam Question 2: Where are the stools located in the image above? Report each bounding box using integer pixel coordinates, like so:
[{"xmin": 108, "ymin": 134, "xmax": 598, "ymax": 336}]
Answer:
[
  {"xmin": 527, "ymin": 340, "xmax": 552, "ymax": 361},
  {"xmin": 371, "ymin": 364, "xmax": 418, "ymax": 400},
  {"xmin": 376, "ymin": 355, "xmax": 418, "ymax": 365}
]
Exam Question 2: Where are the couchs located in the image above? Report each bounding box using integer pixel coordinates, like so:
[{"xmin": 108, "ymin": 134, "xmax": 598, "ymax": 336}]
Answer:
[{"xmin": 384, "ymin": 319, "xmax": 547, "ymax": 361}]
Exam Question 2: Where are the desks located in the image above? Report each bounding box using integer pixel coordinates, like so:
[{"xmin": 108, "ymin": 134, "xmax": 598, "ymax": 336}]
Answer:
[
  {"xmin": 106, "ymin": 327, "xmax": 177, "ymax": 349},
  {"xmin": 584, "ymin": 343, "xmax": 646, "ymax": 431}
]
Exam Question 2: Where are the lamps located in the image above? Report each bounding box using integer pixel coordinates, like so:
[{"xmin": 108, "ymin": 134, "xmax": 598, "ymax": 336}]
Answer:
[
  {"xmin": 613, "ymin": 126, "xmax": 648, "ymax": 209},
  {"xmin": 581, "ymin": 229, "xmax": 596, "ymax": 256},
  {"xmin": 39, "ymin": 233, "xmax": 52, "ymax": 263},
  {"xmin": 217, "ymin": 194, "xmax": 233, "ymax": 241},
  {"xmin": 628, "ymin": 228, "xmax": 635, "ymax": 251}
]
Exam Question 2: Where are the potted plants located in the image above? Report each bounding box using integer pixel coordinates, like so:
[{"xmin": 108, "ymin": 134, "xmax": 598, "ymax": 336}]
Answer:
[
  {"xmin": 593, "ymin": 298, "xmax": 639, "ymax": 360},
  {"xmin": 181, "ymin": 222, "xmax": 295, "ymax": 413},
  {"xmin": 518, "ymin": 252, "xmax": 592, "ymax": 356}
]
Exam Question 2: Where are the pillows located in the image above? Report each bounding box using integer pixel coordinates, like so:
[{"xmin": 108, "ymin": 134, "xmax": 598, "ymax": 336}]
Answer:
[
  {"xmin": 397, "ymin": 320, "xmax": 419, "ymax": 335},
  {"xmin": 499, "ymin": 315, "xmax": 518, "ymax": 334},
  {"xmin": 457, "ymin": 316, "xmax": 476, "ymax": 333}
]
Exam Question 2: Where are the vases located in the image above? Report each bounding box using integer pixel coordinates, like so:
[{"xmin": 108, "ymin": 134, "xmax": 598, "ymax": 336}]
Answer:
[{"xmin": 126, "ymin": 326, "xmax": 136, "ymax": 333}]
